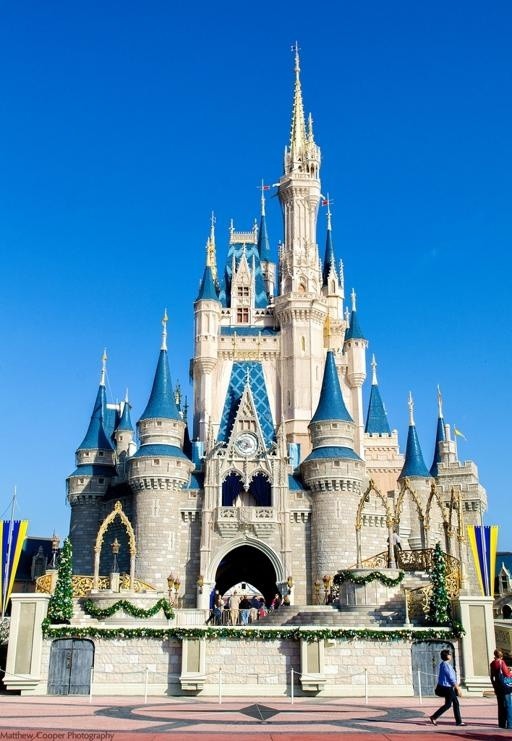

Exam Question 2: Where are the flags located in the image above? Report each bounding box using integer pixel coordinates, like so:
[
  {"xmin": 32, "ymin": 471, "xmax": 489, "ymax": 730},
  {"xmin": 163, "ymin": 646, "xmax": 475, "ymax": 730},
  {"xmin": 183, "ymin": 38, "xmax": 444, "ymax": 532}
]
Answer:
[{"xmin": 454, "ymin": 428, "xmax": 467, "ymax": 441}]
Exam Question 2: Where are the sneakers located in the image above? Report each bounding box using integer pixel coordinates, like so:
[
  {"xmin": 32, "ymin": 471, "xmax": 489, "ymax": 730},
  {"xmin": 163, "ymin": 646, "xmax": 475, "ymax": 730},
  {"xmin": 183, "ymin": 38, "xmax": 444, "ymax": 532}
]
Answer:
[
  {"xmin": 456, "ymin": 722, "xmax": 467, "ymax": 726},
  {"xmin": 430, "ymin": 716, "xmax": 437, "ymax": 726}
]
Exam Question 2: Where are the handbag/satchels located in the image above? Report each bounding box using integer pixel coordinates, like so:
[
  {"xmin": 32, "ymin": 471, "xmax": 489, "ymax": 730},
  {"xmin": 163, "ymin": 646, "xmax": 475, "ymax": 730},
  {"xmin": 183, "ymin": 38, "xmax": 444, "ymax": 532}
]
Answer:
[
  {"xmin": 500, "ymin": 672, "xmax": 512, "ymax": 690},
  {"xmin": 435, "ymin": 683, "xmax": 446, "ymax": 697}
]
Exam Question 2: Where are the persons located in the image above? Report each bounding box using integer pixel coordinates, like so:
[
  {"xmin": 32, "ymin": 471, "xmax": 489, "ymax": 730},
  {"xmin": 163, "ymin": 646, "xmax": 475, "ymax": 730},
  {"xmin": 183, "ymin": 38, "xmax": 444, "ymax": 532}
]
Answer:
[
  {"xmin": 204, "ymin": 589, "xmax": 222, "ymax": 626},
  {"xmin": 489, "ymin": 651, "xmax": 512, "ymax": 731},
  {"xmin": 429, "ymin": 650, "xmax": 467, "ymax": 727},
  {"xmin": 271, "ymin": 593, "xmax": 280, "ymax": 610},
  {"xmin": 215, "ymin": 592, "xmax": 268, "ymax": 625}
]
